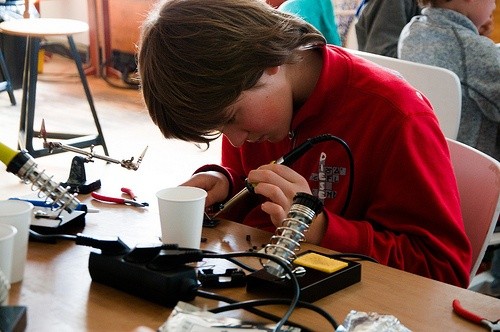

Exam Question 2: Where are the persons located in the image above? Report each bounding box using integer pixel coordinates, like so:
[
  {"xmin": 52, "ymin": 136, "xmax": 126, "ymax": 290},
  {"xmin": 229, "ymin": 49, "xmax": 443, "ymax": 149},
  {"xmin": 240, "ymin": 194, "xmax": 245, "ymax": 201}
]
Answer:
[
  {"xmin": 357, "ymin": 0, "xmax": 419, "ymax": 58},
  {"xmin": 138, "ymin": 0, "xmax": 472, "ymax": 288},
  {"xmin": 278, "ymin": 0, "xmax": 342, "ymax": 46},
  {"xmin": 397, "ymin": 0, "xmax": 500, "ymax": 298}
]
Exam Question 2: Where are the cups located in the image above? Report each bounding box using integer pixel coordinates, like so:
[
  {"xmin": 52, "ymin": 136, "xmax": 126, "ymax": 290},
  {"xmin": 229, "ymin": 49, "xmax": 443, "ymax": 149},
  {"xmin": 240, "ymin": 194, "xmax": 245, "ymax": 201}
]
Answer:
[
  {"xmin": 0, "ymin": 200, "xmax": 34, "ymax": 283},
  {"xmin": 157, "ymin": 186, "xmax": 207, "ymax": 249},
  {"xmin": 0, "ymin": 222, "xmax": 17, "ymax": 290}
]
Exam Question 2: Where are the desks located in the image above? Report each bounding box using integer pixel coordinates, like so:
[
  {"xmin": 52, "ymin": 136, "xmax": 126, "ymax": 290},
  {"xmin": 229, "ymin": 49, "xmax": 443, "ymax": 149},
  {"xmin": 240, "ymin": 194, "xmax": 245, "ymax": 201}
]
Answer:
[
  {"xmin": 0, "ymin": 159, "xmax": 499, "ymax": 332},
  {"xmin": 0, "ymin": 17, "xmax": 110, "ymax": 165}
]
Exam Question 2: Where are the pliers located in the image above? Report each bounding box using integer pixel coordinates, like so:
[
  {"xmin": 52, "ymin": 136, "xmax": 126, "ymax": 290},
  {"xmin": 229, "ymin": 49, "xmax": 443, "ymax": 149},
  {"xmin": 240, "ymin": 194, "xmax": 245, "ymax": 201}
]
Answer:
[
  {"xmin": 91, "ymin": 187, "xmax": 149, "ymax": 207},
  {"xmin": 453, "ymin": 299, "xmax": 500, "ymax": 332}
]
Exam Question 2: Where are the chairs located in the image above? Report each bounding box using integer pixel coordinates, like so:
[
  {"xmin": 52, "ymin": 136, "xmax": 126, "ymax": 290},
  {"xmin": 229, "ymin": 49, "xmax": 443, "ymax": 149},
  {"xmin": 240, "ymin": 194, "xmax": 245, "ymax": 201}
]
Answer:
[{"xmin": 279, "ymin": 0, "xmax": 500, "ymax": 294}]
